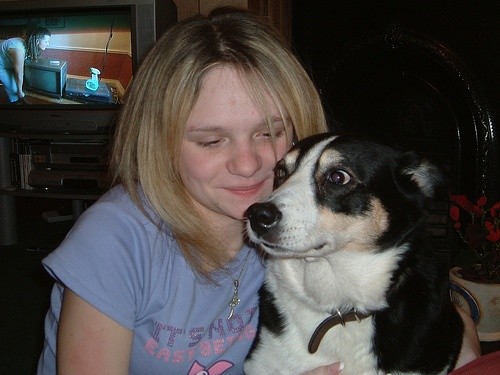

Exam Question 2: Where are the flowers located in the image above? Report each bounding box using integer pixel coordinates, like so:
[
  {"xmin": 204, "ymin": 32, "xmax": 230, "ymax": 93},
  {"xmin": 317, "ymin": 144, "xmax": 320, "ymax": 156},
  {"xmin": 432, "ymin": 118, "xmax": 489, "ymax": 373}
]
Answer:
[{"xmin": 448, "ymin": 192, "xmax": 500, "ymax": 283}]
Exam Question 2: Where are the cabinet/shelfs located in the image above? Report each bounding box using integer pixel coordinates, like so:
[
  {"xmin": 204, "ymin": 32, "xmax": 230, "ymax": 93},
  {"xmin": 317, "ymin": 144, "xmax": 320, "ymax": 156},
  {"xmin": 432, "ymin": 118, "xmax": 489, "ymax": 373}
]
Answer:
[{"xmin": 0, "ymin": 131, "xmax": 115, "ymax": 245}]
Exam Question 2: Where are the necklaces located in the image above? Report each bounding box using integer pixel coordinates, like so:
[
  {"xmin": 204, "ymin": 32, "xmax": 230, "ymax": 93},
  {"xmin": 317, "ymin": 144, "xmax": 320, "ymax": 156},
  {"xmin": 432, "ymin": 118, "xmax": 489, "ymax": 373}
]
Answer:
[{"xmin": 208, "ymin": 243, "xmax": 255, "ymax": 323}]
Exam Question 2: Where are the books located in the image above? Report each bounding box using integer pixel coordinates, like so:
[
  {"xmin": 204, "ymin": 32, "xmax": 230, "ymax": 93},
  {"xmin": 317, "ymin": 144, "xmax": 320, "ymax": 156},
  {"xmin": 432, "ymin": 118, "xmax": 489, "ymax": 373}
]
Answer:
[{"xmin": 10, "ymin": 137, "xmax": 46, "ymax": 192}]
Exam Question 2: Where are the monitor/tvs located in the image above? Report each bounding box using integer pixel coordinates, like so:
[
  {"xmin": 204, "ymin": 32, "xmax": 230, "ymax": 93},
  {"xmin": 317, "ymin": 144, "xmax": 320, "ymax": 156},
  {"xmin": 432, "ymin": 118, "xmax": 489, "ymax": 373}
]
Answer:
[
  {"xmin": 23, "ymin": 56, "xmax": 67, "ymax": 100},
  {"xmin": 0, "ymin": 0, "xmax": 179, "ymax": 142}
]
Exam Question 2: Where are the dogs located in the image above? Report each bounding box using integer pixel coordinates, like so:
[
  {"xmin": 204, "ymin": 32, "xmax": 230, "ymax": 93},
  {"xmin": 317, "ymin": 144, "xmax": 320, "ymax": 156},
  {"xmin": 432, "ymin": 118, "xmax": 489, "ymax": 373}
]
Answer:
[{"xmin": 243, "ymin": 132, "xmax": 465, "ymax": 375}]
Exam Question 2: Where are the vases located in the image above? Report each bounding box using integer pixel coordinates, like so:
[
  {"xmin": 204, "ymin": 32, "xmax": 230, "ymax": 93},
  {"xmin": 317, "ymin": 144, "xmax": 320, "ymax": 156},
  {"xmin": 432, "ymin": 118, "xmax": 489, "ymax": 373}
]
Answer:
[{"xmin": 450, "ymin": 264, "xmax": 500, "ymax": 342}]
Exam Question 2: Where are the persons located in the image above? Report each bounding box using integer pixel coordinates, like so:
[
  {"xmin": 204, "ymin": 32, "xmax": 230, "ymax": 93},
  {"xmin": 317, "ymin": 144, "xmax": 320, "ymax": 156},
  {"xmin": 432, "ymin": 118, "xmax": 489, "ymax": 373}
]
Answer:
[
  {"xmin": 0, "ymin": 27, "xmax": 52, "ymax": 103},
  {"xmin": 38, "ymin": 8, "xmax": 481, "ymax": 375}
]
